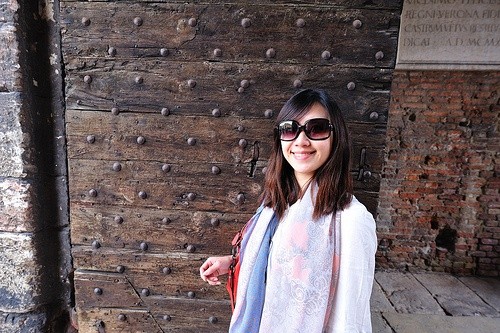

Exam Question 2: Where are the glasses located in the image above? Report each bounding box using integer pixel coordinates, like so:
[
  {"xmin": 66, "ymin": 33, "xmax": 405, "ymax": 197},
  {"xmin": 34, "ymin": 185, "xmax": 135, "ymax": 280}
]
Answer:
[{"xmin": 278, "ymin": 118, "xmax": 334, "ymax": 141}]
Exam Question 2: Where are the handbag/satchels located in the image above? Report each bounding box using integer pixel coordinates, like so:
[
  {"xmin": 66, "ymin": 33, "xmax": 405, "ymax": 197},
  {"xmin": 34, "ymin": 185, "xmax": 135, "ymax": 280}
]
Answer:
[{"xmin": 226, "ymin": 226, "xmax": 246, "ymax": 313}]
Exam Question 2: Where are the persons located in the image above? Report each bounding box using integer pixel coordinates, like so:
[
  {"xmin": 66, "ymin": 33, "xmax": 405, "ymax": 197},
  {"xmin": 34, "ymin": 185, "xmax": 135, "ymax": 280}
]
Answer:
[{"xmin": 200, "ymin": 88, "xmax": 378, "ymax": 333}]
